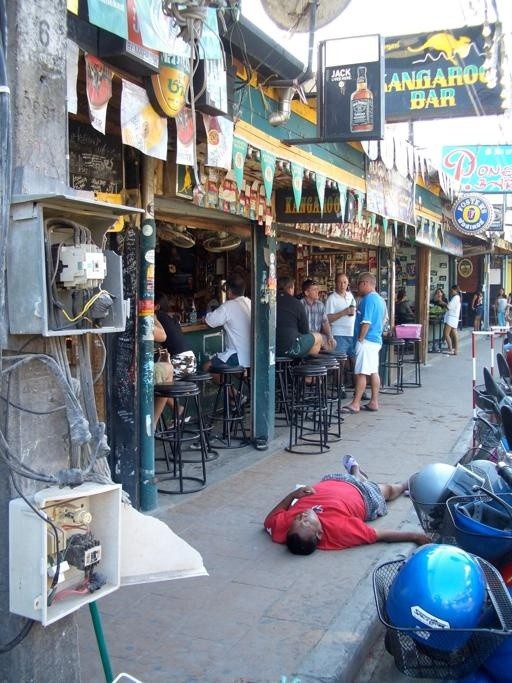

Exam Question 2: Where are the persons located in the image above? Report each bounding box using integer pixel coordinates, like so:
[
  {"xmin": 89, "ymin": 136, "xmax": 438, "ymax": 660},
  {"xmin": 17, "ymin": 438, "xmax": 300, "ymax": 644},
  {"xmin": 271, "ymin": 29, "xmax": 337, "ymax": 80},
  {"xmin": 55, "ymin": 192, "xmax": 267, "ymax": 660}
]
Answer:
[
  {"xmin": 505, "ymin": 293, "xmax": 512, "ymax": 332},
  {"xmin": 393, "ymin": 287, "xmax": 419, "ymax": 327},
  {"xmin": 325, "ymin": 273, "xmax": 369, "ymax": 413},
  {"xmin": 503, "ymin": 327, "xmax": 512, "ymax": 385},
  {"xmin": 495, "ymin": 289, "xmax": 508, "ymax": 327},
  {"xmin": 277, "ymin": 277, "xmax": 323, "ymax": 385},
  {"xmin": 473, "ymin": 284, "xmax": 484, "ymax": 331},
  {"xmin": 441, "ymin": 282, "xmax": 462, "ymax": 355},
  {"xmin": 430, "ymin": 289, "xmax": 448, "ymax": 308},
  {"xmin": 336, "ymin": 272, "xmax": 386, "ymax": 413},
  {"xmin": 154, "ymin": 293, "xmax": 197, "ymax": 428},
  {"xmin": 203, "ymin": 274, "xmax": 251, "ymax": 411},
  {"xmin": 299, "ymin": 280, "xmax": 336, "ymax": 383},
  {"xmin": 155, "ymin": 308, "xmax": 174, "ymax": 435},
  {"xmin": 263, "ymin": 453, "xmax": 432, "ymax": 554}
]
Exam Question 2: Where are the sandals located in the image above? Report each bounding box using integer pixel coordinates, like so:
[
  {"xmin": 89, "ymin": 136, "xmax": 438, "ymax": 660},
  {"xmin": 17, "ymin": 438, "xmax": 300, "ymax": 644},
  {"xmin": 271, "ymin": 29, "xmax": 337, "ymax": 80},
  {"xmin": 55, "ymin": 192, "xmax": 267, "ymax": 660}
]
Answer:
[
  {"xmin": 255, "ymin": 435, "xmax": 268, "ymax": 450},
  {"xmin": 231, "ymin": 391, "xmax": 247, "ymax": 411},
  {"xmin": 434, "ymin": 343, "xmax": 440, "ymax": 353},
  {"xmin": 429, "ymin": 343, "xmax": 433, "ymax": 352}
]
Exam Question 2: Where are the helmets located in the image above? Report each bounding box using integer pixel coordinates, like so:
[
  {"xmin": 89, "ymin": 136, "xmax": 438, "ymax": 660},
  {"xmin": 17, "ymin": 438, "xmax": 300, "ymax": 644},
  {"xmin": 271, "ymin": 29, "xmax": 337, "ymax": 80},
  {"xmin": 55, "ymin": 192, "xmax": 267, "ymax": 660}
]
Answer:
[
  {"xmin": 453, "ymin": 499, "xmax": 512, "ymax": 537},
  {"xmin": 386, "ymin": 543, "xmax": 487, "ymax": 653},
  {"xmin": 411, "ymin": 463, "xmax": 456, "ymax": 519},
  {"xmin": 463, "ymin": 459, "xmax": 512, "ymax": 523}
]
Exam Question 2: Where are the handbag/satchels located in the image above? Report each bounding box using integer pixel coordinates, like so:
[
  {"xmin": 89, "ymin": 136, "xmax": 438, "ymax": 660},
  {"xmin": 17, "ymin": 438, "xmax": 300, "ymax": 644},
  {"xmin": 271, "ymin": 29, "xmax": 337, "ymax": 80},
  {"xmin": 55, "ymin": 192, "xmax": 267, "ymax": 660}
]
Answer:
[{"xmin": 154, "ymin": 348, "xmax": 174, "ymax": 384}]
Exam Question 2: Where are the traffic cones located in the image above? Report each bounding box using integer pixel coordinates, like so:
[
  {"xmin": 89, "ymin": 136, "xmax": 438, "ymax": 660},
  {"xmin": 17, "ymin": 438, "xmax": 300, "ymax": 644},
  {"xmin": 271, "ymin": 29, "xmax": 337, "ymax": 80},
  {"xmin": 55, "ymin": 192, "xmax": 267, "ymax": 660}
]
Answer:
[{"xmin": 472, "ymin": 329, "xmax": 495, "ymax": 455}]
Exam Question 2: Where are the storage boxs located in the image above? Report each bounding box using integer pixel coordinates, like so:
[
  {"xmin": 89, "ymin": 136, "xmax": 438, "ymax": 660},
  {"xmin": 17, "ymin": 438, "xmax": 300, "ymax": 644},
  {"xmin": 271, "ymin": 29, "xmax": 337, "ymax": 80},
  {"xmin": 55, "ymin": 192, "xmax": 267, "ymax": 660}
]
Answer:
[{"xmin": 395, "ymin": 324, "xmax": 423, "ymax": 338}]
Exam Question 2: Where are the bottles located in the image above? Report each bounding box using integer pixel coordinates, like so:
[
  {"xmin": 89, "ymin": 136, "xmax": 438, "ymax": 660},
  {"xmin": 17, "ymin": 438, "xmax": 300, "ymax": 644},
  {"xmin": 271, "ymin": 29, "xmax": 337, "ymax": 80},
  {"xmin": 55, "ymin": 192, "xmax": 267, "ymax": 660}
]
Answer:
[
  {"xmin": 350, "ymin": 67, "xmax": 374, "ymax": 132},
  {"xmin": 179, "ymin": 297, "xmax": 187, "ymax": 323},
  {"xmin": 189, "ymin": 299, "xmax": 198, "ymax": 325}
]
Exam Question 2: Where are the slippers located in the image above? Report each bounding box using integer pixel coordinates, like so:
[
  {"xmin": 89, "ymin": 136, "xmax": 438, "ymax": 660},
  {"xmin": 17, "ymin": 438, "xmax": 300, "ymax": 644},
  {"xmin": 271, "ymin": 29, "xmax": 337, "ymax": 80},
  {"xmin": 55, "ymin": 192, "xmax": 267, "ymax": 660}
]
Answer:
[
  {"xmin": 360, "ymin": 405, "xmax": 378, "ymax": 411},
  {"xmin": 452, "ymin": 349, "xmax": 458, "ymax": 355},
  {"xmin": 337, "ymin": 406, "xmax": 360, "ymax": 413},
  {"xmin": 443, "ymin": 351, "xmax": 454, "ymax": 354},
  {"xmin": 165, "ymin": 416, "xmax": 192, "ymax": 429},
  {"xmin": 343, "ymin": 454, "xmax": 368, "ymax": 480}
]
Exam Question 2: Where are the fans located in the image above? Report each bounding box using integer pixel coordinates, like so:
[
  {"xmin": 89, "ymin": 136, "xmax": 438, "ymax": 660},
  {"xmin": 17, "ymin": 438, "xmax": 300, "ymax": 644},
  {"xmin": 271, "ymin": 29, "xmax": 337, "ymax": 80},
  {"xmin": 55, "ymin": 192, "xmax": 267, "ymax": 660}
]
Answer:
[
  {"xmin": 203, "ymin": 229, "xmax": 242, "ymax": 252},
  {"xmin": 156, "ymin": 223, "xmax": 196, "ymax": 248}
]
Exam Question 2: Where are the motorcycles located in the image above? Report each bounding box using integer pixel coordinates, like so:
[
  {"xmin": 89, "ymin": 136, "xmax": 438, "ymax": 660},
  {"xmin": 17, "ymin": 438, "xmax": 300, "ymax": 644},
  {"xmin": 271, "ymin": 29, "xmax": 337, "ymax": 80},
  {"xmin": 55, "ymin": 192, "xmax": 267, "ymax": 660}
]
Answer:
[
  {"xmin": 455, "ymin": 352, "xmax": 512, "ymax": 474},
  {"xmin": 408, "ymin": 460, "xmax": 512, "ymax": 543},
  {"xmin": 443, "ymin": 485, "xmax": 512, "ymax": 562},
  {"xmin": 373, "ymin": 543, "xmax": 512, "ymax": 683}
]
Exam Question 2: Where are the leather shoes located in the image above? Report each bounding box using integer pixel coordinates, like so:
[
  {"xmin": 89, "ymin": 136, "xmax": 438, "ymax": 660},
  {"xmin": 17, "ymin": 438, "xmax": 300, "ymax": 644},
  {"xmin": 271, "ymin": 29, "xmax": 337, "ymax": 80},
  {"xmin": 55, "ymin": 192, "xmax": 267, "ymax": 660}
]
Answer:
[
  {"xmin": 342, "ymin": 391, "xmax": 347, "ymax": 399},
  {"xmin": 353, "ymin": 392, "xmax": 370, "ymax": 400}
]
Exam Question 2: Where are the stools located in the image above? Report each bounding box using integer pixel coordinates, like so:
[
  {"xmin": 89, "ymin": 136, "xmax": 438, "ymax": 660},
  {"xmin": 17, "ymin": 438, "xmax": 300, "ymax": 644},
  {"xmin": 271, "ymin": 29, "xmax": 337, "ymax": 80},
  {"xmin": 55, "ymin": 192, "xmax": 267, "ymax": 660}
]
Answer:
[
  {"xmin": 275, "ymin": 356, "xmax": 296, "ymax": 428},
  {"xmin": 305, "ymin": 350, "xmax": 348, "ymax": 424},
  {"xmin": 378, "ymin": 336, "xmax": 406, "ymax": 395},
  {"xmin": 284, "ymin": 368, "xmax": 332, "ymax": 455},
  {"xmin": 154, "ymin": 380, "xmax": 208, "ymax": 494},
  {"xmin": 298, "ymin": 357, "xmax": 343, "ymax": 442},
  {"xmin": 208, "ymin": 365, "xmax": 251, "ymax": 449},
  {"xmin": 168, "ymin": 372, "xmax": 220, "ymax": 462},
  {"xmin": 394, "ymin": 337, "xmax": 422, "ymax": 388}
]
